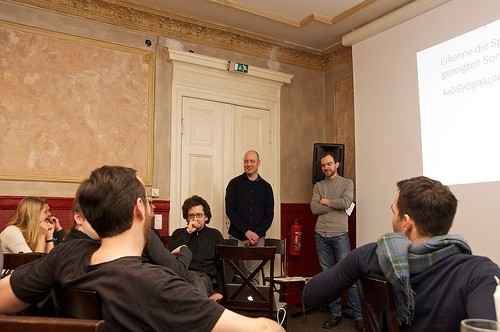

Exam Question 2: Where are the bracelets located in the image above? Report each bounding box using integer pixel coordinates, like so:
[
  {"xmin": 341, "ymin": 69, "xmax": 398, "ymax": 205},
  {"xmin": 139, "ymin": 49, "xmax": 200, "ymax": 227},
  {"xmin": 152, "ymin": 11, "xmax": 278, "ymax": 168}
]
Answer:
[{"xmin": 46, "ymin": 238, "xmax": 54, "ymax": 243}]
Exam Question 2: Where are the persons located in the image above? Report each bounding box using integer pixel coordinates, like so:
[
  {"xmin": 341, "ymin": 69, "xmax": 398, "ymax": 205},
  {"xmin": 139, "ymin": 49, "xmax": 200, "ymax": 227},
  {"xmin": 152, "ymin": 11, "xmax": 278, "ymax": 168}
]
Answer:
[
  {"xmin": 226, "ymin": 150, "xmax": 274, "ymax": 284},
  {"xmin": 311, "ymin": 151, "xmax": 366, "ymax": 331},
  {"xmin": 302, "ymin": 176, "xmax": 500, "ymax": 331},
  {"xmin": 165, "ymin": 195, "xmax": 226, "ymax": 302},
  {"xmin": 1, "ymin": 196, "xmax": 56, "ymax": 278},
  {"xmin": 42, "ymin": 198, "xmax": 65, "ymax": 246},
  {"xmin": 141, "ymin": 198, "xmax": 192, "ymax": 274},
  {"xmin": 0, "ymin": 165, "xmax": 286, "ymax": 332},
  {"xmin": 61, "ymin": 198, "xmax": 102, "ymax": 243}
]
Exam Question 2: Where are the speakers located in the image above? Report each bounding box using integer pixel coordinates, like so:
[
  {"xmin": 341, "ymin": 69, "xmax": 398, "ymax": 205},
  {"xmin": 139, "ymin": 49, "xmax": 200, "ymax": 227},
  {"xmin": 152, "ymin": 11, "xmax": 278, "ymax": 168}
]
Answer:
[{"xmin": 312, "ymin": 143, "xmax": 344, "ymax": 184}]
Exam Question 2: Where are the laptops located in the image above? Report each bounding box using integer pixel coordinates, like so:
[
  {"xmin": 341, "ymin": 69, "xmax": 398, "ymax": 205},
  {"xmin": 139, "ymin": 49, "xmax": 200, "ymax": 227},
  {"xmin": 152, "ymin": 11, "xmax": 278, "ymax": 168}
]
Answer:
[{"xmin": 227, "ymin": 283, "xmax": 287, "ymax": 312}]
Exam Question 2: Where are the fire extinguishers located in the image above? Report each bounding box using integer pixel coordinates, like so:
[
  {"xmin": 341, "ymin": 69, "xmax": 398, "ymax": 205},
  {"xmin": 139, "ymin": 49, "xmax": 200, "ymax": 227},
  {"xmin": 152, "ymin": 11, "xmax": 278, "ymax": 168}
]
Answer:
[{"xmin": 288, "ymin": 217, "xmax": 303, "ymax": 257}]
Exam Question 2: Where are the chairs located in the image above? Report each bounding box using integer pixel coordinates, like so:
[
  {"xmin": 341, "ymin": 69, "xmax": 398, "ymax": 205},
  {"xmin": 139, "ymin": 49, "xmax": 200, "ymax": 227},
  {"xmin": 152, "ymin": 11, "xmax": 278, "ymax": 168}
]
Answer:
[
  {"xmin": 0, "ymin": 314, "xmax": 105, "ymax": 332},
  {"xmin": 356, "ymin": 275, "xmax": 401, "ymax": 332},
  {"xmin": 265, "ymin": 238, "xmax": 309, "ymax": 325},
  {"xmin": 3, "ymin": 252, "xmax": 46, "ymax": 277},
  {"xmin": 215, "ymin": 245, "xmax": 288, "ymax": 332}
]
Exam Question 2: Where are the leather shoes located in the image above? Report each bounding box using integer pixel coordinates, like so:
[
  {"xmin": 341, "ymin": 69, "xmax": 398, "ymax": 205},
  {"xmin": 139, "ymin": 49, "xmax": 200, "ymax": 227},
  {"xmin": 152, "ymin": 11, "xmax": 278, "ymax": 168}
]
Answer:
[
  {"xmin": 323, "ymin": 315, "xmax": 343, "ymax": 329},
  {"xmin": 354, "ymin": 319, "xmax": 366, "ymax": 332}
]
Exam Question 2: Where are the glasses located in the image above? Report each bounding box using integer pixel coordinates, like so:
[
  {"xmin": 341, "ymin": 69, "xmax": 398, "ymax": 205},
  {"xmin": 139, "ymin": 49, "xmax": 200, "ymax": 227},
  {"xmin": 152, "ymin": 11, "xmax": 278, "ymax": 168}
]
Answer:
[{"xmin": 187, "ymin": 213, "xmax": 205, "ymax": 219}]
